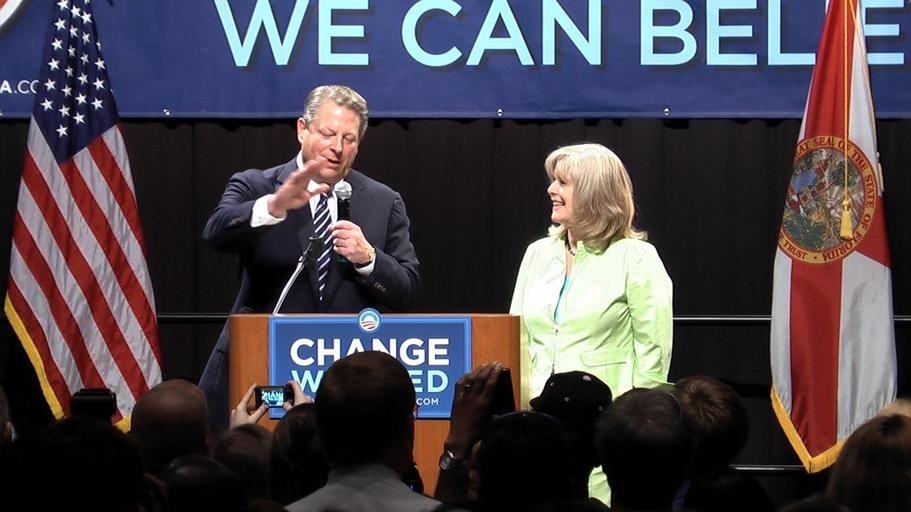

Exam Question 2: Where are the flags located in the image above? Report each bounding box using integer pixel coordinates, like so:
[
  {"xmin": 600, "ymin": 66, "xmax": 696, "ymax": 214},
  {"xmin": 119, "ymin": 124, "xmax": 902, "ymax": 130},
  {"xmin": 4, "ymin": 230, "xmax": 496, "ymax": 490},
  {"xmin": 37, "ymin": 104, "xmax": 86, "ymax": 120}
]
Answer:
[
  {"xmin": 767, "ymin": 0, "xmax": 899, "ymax": 478},
  {"xmin": 3, "ymin": 0, "xmax": 163, "ymax": 436}
]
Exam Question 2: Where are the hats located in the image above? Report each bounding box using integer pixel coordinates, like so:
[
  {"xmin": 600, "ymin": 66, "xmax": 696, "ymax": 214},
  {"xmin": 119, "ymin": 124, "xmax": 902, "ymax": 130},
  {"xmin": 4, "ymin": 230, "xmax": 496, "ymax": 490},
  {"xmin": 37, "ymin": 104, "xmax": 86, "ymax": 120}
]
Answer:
[{"xmin": 529, "ymin": 370, "xmax": 613, "ymax": 429}]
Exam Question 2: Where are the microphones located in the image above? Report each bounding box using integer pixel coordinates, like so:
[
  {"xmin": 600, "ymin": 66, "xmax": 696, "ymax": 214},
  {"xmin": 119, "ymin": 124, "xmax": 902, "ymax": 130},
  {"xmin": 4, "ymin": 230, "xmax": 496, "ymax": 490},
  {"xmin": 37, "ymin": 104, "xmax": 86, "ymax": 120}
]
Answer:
[{"xmin": 334, "ymin": 182, "xmax": 353, "ymax": 263}]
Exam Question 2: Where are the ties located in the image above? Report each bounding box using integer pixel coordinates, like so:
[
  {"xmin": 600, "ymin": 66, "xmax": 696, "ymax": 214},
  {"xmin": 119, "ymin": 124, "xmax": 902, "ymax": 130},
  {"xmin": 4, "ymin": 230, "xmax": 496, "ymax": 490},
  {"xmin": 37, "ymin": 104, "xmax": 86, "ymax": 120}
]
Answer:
[{"xmin": 311, "ymin": 185, "xmax": 336, "ymax": 307}]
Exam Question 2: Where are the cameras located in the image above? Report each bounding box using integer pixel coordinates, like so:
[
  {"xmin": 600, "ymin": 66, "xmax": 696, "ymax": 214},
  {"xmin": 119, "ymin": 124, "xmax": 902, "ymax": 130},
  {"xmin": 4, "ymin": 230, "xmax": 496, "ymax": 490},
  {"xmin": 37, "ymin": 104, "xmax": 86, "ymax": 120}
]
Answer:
[{"xmin": 255, "ymin": 386, "xmax": 294, "ymax": 408}]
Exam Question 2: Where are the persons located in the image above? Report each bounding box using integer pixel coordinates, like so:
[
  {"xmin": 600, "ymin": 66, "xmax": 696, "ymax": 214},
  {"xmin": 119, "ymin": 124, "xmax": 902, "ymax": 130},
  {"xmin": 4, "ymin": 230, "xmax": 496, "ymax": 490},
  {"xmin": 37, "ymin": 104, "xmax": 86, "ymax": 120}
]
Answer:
[
  {"xmin": 196, "ymin": 82, "xmax": 420, "ymax": 455},
  {"xmin": 504, "ymin": 140, "xmax": 674, "ymax": 508},
  {"xmin": 1, "ymin": 349, "xmax": 911, "ymax": 512}
]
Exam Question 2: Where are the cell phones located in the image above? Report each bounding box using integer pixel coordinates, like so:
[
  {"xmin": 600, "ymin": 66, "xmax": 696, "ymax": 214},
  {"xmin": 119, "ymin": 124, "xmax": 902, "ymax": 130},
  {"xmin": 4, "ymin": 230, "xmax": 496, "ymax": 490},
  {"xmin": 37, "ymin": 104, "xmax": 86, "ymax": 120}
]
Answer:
[{"xmin": 489, "ymin": 368, "xmax": 518, "ymax": 419}]
